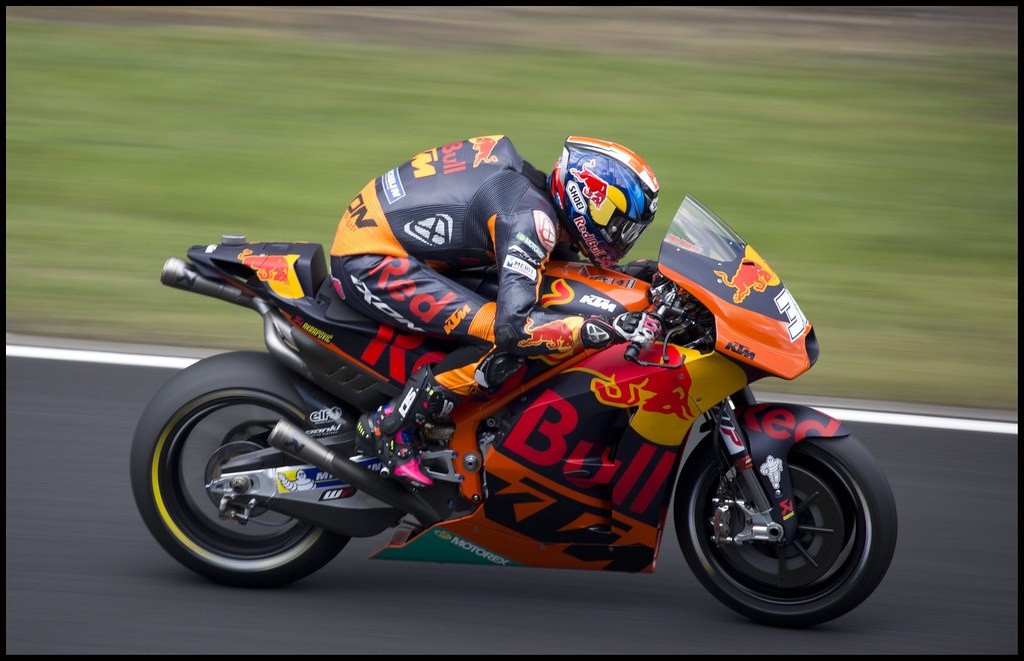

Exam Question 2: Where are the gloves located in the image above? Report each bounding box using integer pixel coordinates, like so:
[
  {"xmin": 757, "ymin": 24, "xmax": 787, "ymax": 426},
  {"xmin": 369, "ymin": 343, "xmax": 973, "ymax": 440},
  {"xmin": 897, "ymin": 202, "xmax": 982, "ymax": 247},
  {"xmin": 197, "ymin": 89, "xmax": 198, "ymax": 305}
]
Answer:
[{"xmin": 578, "ymin": 311, "xmax": 662, "ymax": 351}]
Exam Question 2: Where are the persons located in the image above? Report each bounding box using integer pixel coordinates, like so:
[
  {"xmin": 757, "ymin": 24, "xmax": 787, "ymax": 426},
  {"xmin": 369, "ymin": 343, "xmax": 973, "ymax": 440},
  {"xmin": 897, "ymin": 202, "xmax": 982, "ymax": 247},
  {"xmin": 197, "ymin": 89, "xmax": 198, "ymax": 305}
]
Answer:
[{"xmin": 329, "ymin": 136, "xmax": 659, "ymax": 486}]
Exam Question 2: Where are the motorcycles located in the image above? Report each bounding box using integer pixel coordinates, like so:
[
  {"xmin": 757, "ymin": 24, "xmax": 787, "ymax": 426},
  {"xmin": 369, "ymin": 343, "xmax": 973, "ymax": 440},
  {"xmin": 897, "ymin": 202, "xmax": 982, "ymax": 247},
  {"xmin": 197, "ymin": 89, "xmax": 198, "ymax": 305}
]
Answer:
[{"xmin": 128, "ymin": 192, "xmax": 900, "ymax": 627}]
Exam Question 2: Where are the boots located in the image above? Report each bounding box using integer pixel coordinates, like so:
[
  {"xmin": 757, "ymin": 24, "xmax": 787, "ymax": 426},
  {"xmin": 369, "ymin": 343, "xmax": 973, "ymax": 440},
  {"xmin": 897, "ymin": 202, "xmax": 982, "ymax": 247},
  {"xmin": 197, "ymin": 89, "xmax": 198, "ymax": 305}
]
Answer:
[{"xmin": 355, "ymin": 360, "xmax": 455, "ymax": 485}]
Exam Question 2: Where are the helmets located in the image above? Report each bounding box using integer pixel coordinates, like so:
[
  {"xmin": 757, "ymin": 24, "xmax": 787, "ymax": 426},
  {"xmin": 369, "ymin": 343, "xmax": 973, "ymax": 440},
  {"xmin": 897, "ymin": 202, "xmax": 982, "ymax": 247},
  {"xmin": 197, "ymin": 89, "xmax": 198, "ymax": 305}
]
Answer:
[{"xmin": 547, "ymin": 135, "xmax": 661, "ymax": 269}]
